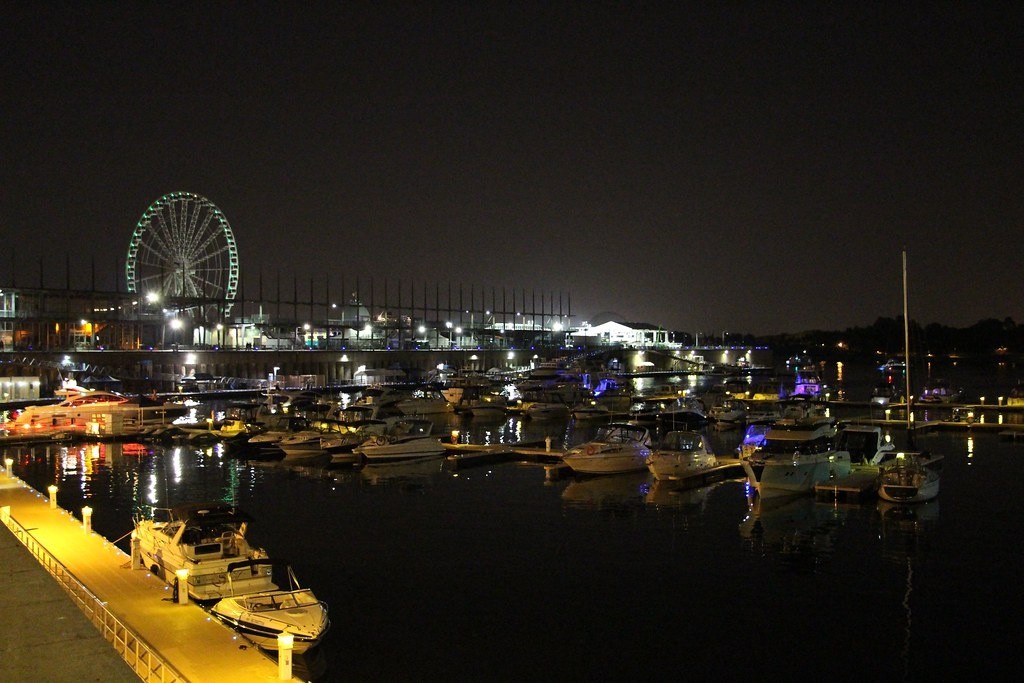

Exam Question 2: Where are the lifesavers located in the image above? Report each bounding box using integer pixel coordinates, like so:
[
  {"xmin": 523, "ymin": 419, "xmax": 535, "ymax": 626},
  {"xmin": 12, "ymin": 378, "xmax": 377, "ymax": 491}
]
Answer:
[
  {"xmin": 587, "ymin": 446, "xmax": 594, "ymax": 456},
  {"xmin": 451, "ymin": 436, "xmax": 456, "ymax": 442},
  {"xmin": 792, "ymin": 450, "xmax": 801, "ymax": 463}
]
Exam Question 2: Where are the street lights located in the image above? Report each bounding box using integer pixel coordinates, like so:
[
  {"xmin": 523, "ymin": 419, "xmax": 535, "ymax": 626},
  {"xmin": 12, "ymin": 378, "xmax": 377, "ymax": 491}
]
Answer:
[
  {"xmin": 517, "ymin": 312, "xmax": 525, "ymax": 350},
  {"xmin": 445, "ymin": 322, "xmax": 452, "ymax": 348},
  {"xmin": 466, "ymin": 310, "xmax": 474, "ymax": 349},
  {"xmin": 485, "ymin": 311, "xmax": 495, "ymax": 348},
  {"xmin": 332, "ymin": 303, "xmax": 344, "ymax": 349},
  {"xmin": 148, "ymin": 292, "xmax": 166, "ymax": 351}
]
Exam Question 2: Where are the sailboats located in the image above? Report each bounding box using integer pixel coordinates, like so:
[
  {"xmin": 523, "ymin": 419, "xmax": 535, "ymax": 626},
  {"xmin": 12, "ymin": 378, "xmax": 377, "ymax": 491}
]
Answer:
[{"xmin": 879, "ymin": 250, "xmax": 941, "ymax": 504}]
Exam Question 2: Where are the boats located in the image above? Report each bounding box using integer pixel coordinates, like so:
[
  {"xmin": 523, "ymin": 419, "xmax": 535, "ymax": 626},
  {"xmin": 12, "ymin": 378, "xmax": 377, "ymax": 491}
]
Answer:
[
  {"xmin": 130, "ymin": 456, "xmax": 331, "ymax": 656},
  {"xmin": 0, "ymin": 244, "xmax": 892, "ymax": 501}
]
